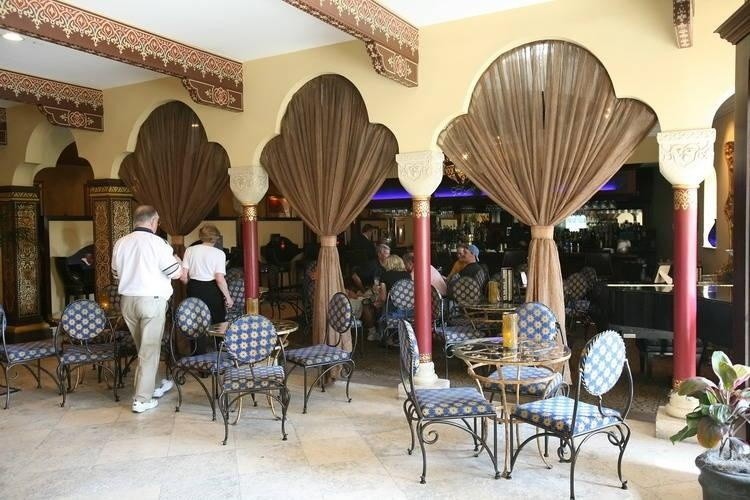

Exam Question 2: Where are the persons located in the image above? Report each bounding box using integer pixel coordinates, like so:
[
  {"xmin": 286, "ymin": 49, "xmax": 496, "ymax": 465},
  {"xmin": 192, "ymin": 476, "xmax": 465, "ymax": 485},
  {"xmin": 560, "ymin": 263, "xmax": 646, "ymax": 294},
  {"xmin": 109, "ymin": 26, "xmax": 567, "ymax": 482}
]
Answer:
[
  {"xmin": 110, "ymin": 205, "xmax": 183, "ymax": 413},
  {"xmin": 310, "ymin": 224, "xmax": 531, "ymax": 347},
  {"xmin": 180, "ymin": 225, "xmax": 233, "ymax": 378}
]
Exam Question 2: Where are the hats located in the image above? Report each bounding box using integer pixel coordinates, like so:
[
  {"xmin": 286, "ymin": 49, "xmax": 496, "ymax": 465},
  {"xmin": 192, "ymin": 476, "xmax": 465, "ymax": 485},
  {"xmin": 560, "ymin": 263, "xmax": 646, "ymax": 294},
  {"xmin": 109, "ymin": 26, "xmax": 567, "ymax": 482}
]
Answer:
[{"xmin": 466, "ymin": 245, "xmax": 480, "ymax": 261}]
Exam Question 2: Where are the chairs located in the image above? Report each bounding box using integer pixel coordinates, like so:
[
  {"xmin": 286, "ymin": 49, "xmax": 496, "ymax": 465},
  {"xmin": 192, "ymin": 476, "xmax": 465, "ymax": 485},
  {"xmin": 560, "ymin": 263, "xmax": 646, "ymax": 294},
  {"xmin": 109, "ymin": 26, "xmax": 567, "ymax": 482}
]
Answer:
[
  {"xmin": 299, "ymin": 255, "xmax": 599, "ymax": 373},
  {"xmin": 0, "ymin": 304, "xmax": 64, "ymax": 410},
  {"xmin": 97, "ymin": 254, "xmax": 293, "ymax": 381},
  {"xmin": 503, "ymin": 330, "xmax": 634, "ymax": 500},
  {"xmin": 397, "ymin": 319, "xmax": 502, "ymax": 485},
  {"xmin": 55, "ymin": 299, "xmax": 121, "ymax": 407},
  {"xmin": 279, "ymin": 291, "xmax": 357, "ymax": 414},
  {"xmin": 217, "ymin": 313, "xmax": 292, "ymax": 446},
  {"xmin": 479, "ymin": 301, "xmax": 567, "ymax": 458},
  {"xmin": 168, "ymin": 296, "xmax": 259, "ymax": 422}
]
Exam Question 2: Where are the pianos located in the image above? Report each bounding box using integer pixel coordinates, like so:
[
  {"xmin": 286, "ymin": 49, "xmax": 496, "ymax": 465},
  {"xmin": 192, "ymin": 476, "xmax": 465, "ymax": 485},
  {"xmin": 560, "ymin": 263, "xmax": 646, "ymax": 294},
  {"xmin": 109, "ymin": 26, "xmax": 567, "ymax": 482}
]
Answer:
[{"xmin": 593, "ymin": 282, "xmax": 734, "ymax": 376}]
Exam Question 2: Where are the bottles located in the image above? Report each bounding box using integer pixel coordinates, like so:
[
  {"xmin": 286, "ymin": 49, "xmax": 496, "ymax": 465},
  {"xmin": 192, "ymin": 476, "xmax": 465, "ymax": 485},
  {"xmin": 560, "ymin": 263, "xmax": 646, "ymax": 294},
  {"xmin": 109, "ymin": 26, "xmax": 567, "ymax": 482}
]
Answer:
[
  {"xmin": 563, "ymin": 229, "xmax": 584, "ymax": 250},
  {"xmin": 439, "ymin": 224, "xmax": 456, "ymax": 239},
  {"xmin": 621, "ymin": 220, "xmax": 642, "ymax": 232}
]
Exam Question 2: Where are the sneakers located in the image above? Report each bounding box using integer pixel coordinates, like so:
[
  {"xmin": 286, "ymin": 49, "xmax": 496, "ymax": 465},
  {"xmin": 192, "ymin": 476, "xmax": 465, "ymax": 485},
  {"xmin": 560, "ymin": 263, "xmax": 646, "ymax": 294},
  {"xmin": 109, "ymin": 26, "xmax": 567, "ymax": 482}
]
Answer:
[
  {"xmin": 366, "ymin": 331, "xmax": 381, "ymax": 341},
  {"xmin": 153, "ymin": 379, "xmax": 173, "ymax": 397},
  {"xmin": 132, "ymin": 398, "xmax": 158, "ymax": 413}
]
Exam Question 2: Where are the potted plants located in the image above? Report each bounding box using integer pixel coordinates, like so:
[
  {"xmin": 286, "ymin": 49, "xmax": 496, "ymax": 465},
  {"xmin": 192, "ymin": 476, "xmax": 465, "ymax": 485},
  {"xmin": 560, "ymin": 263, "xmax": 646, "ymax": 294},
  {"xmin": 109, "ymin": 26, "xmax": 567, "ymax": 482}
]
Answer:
[{"xmin": 668, "ymin": 349, "xmax": 749, "ymax": 500}]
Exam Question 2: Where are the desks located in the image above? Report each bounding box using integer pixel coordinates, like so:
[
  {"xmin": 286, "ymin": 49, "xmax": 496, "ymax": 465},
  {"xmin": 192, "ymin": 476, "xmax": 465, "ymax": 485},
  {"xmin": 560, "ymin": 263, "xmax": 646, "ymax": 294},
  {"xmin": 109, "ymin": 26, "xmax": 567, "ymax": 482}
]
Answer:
[
  {"xmin": 451, "ymin": 336, "xmax": 571, "ymax": 477},
  {"xmin": 47, "ymin": 308, "xmax": 125, "ymax": 392},
  {"xmin": 208, "ymin": 316, "xmax": 299, "ymax": 424}
]
